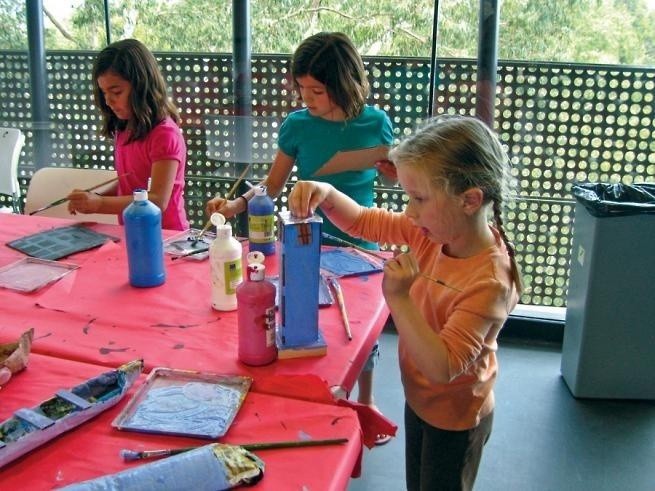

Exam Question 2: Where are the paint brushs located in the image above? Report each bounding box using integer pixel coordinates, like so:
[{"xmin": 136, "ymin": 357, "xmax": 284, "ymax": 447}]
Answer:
[
  {"xmin": 190, "ymin": 162, "xmax": 252, "ymax": 248},
  {"xmin": 330, "ymin": 277, "xmax": 352, "ymax": 339},
  {"xmin": 120, "ymin": 437, "xmax": 350, "ymax": 461},
  {"xmin": 29, "ymin": 172, "xmax": 131, "ymax": 215},
  {"xmin": 171, "ymin": 237, "xmax": 248, "ymax": 260},
  {"xmin": 320, "ymin": 231, "xmax": 463, "ymax": 291}
]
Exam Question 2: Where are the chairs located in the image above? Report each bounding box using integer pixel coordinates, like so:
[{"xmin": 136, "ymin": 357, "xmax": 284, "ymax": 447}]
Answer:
[
  {"xmin": 24, "ymin": 168, "xmax": 121, "ymax": 225},
  {"xmin": 0, "ymin": 127, "xmax": 28, "ymax": 213}
]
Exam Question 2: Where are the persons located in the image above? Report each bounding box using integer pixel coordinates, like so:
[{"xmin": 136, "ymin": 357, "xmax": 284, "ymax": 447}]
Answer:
[
  {"xmin": 287, "ymin": 113, "xmax": 522, "ymax": 490},
  {"xmin": 206, "ymin": 30, "xmax": 400, "ymax": 446},
  {"xmin": 66, "ymin": 38, "xmax": 190, "ymax": 231}
]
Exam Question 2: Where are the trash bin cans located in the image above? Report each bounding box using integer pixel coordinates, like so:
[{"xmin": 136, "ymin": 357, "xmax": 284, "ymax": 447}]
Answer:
[{"xmin": 561, "ymin": 183, "xmax": 655, "ymax": 400}]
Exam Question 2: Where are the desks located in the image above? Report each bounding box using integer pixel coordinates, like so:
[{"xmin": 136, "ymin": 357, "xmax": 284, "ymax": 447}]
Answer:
[
  {"xmin": 0, "ymin": 210, "xmax": 401, "ymax": 405},
  {"xmin": 0, "ymin": 343, "xmax": 368, "ymax": 491}
]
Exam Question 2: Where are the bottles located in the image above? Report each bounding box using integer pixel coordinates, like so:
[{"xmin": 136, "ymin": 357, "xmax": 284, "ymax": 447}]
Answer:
[
  {"xmin": 244, "ymin": 179, "xmax": 276, "ymax": 257},
  {"xmin": 209, "ymin": 211, "xmax": 244, "ymax": 312},
  {"xmin": 122, "ymin": 189, "xmax": 166, "ymax": 288},
  {"xmin": 235, "ymin": 250, "xmax": 277, "ymax": 367}
]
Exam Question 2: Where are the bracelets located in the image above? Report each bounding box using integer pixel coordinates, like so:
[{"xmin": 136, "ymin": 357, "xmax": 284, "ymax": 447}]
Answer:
[{"xmin": 238, "ymin": 195, "xmax": 248, "ymax": 211}]
